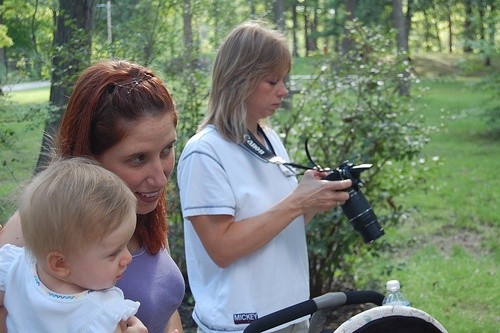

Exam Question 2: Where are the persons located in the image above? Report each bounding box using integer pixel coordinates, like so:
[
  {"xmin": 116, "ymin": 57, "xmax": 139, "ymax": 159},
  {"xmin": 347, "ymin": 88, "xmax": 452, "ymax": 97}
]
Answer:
[
  {"xmin": 177, "ymin": 22, "xmax": 352, "ymax": 333},
  {"xmin": 0, "ymin": 61, "xmax": 185, "ymax": 333},
  {"xmin": 0, "ymin": 159, "xmax": 149, "ymax": 333}
]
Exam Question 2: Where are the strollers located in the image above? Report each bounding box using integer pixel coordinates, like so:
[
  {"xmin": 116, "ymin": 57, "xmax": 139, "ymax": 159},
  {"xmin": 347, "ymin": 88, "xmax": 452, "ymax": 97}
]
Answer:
[{"xmin": 243, "ymin": 291, "xmax": 447, "ymax": 333}]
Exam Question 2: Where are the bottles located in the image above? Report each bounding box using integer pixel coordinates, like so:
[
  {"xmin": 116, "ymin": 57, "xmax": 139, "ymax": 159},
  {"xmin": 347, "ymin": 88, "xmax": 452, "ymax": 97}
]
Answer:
[{"xmin": 382, "ymin": 280, "xmax": 410, "ymax": 307}]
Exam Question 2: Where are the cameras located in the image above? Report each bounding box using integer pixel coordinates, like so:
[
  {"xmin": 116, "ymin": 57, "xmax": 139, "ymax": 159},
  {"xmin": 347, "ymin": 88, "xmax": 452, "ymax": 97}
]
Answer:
[{"xmin": 320, "ymin": 160, "xmax": 385, "ymax": 243}]
